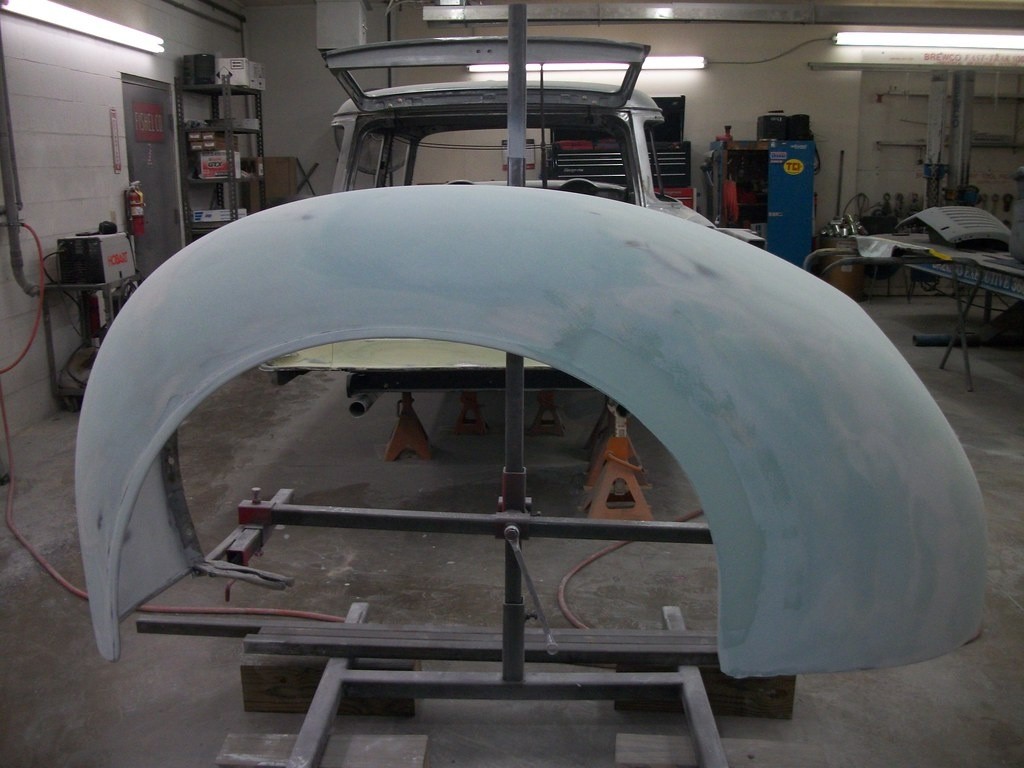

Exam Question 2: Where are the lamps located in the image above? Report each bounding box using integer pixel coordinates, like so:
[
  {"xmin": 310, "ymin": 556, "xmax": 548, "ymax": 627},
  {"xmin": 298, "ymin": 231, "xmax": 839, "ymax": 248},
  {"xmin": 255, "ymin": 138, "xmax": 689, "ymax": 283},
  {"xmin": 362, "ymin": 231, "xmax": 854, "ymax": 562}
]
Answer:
[
  {"xmin": 1, "ymin": 0, "xmax": 165, "ymax": 54},
  {"xmin": 466, "ymin": 55, "xmax": 708, "ymax": 74},
  {"xmin": 832, "ymin": 31, "xmax": 1024, "ymax": 49}
]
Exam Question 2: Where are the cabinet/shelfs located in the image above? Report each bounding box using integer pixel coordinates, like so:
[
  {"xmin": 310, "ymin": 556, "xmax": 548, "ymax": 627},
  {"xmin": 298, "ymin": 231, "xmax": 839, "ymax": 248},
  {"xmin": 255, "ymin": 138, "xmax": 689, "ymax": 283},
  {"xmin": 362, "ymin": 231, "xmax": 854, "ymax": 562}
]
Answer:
[
  {"xmin": 172, "ymin": 74, "xmax": 264, "ymax": 246},
  {"xmin": 873, "ymin": 91, "xmax": 1024, "ymax": 149},
  {"xmin": 41, "ymin": 272, "xmax": 143, "ymax": 399},
  {"xmin": 712, "ymin": 136, "xmax": 816, "ymax": 271}
]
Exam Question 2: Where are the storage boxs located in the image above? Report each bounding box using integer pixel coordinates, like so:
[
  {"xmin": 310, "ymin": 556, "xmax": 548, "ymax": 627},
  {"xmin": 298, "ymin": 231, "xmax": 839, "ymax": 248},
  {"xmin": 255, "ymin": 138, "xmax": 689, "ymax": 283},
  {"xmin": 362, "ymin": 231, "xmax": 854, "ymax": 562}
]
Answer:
[
  {"xmin": 195, "ymin": 150, "xmax": 243, "ymax": 179},
  {"xmin": 213, "ymin": 57, "xmax": 249, "ymax": 86},
  {"xmin": 241, "ymin": 153, "xmax": 298, "ymax": 197},
  {"xmin": 187, "ymin": 130, "xmax": 239, "ymax": 151},
  {"xmin": 247, "ymin": 59, "xmax": 264, "ymax": 90},
  {"xmin": 188, "ymin": 207, "xmax": 246, "ymax": 223}
]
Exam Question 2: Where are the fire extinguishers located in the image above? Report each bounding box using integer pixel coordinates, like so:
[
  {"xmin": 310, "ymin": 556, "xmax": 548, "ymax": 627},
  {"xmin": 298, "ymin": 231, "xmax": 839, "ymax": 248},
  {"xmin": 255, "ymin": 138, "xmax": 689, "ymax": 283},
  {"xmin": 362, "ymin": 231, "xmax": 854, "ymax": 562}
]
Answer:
[{"xmin": 125, "ymin": 180, "xmax": 146, "ymax": 236}]
